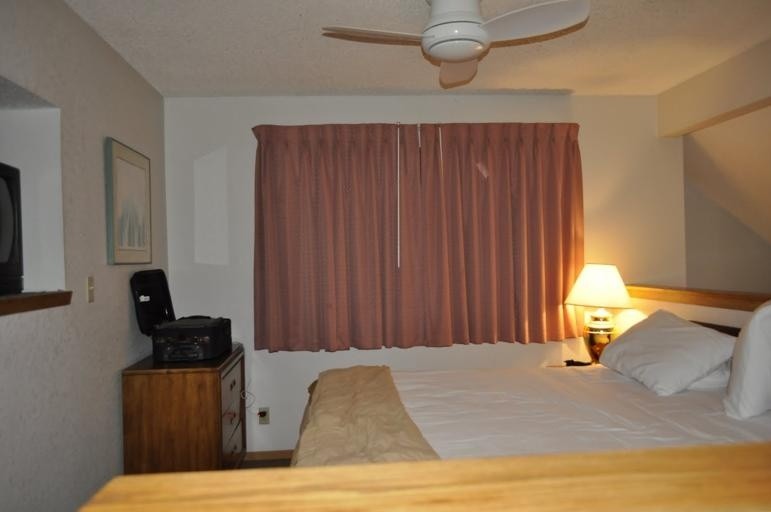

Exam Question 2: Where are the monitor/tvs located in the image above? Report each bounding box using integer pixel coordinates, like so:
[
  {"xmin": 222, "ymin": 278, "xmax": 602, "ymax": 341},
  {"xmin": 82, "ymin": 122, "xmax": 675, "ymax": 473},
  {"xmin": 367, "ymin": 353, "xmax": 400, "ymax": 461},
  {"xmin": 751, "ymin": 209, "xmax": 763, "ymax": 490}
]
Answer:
[{"xmin": 0, "ymin": 163, "xmax": 24, "ymax": 296}]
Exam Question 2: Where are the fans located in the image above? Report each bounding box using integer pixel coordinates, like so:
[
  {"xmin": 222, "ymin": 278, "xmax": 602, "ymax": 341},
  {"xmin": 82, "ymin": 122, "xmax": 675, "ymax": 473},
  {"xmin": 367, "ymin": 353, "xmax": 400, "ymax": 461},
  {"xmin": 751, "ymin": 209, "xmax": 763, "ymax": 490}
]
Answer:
[{"xmin": 321, "ymin": 2, "xmax": 590, "ymax": 86}]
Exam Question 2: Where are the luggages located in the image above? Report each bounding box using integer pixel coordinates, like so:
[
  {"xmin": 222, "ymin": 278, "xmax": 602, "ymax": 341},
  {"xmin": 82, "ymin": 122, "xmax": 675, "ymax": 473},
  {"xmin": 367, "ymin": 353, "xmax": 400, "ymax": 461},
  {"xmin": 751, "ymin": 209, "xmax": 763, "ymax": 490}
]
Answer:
[{"xmin": 130, "ymin": 268, "xmax": 233, "ymax": 368}]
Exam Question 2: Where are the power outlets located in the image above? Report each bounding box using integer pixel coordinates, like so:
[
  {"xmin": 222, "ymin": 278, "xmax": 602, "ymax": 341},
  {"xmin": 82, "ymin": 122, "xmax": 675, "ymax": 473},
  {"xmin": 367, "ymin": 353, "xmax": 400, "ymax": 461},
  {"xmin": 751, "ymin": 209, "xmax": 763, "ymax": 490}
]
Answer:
[{"xmin": 257, "ymin": 406, "xmax": 271, "ymax": 426}]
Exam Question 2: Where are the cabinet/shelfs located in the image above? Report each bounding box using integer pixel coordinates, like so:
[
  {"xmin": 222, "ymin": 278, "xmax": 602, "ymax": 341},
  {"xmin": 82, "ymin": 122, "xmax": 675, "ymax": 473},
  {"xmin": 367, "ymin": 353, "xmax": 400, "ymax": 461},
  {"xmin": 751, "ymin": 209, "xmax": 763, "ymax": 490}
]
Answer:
[{"xmin": 120, "ymin": 342, "xmax": 247, "ymax": 473}]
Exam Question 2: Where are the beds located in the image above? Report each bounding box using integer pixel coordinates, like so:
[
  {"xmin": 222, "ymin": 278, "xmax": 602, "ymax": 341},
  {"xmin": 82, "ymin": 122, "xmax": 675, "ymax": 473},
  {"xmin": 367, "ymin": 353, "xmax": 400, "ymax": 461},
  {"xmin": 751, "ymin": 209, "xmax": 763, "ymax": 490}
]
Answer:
[{"xmin": 292, "ymin": 321, "xmax": 770, "ymax": 468}]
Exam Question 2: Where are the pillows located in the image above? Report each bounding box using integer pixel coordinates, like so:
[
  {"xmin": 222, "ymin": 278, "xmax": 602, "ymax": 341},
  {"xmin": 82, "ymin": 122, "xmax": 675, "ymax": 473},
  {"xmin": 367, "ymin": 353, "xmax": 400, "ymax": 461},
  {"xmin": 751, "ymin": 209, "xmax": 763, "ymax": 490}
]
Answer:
[{"xmin": 599, "ymin": 301, "xmax": 770, "ymax": 422}]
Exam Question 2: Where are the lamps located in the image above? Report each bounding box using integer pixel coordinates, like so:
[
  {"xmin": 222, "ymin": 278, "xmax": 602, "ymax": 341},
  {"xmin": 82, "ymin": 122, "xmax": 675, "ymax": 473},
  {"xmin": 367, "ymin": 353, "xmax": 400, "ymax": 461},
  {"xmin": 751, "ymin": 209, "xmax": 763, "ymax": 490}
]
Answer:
[{"xmin": 562, "ymin": 263, "xmax": 630, "ymax": 362}]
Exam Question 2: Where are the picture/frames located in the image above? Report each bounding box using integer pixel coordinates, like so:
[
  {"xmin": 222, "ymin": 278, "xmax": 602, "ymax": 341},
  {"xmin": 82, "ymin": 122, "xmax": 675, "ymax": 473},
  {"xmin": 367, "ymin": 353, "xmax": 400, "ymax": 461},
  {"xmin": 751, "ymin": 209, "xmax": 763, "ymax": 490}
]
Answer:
[{"xmin": 104, "ymin": 134, "xmax": 151, "ymax": 267}]
